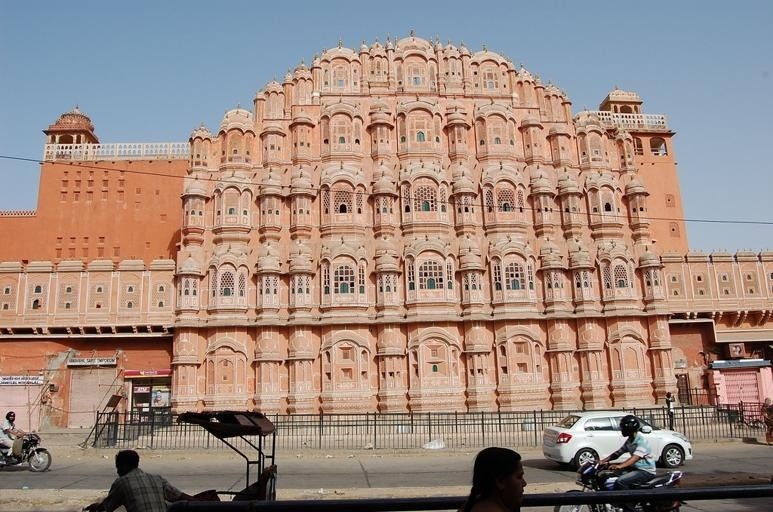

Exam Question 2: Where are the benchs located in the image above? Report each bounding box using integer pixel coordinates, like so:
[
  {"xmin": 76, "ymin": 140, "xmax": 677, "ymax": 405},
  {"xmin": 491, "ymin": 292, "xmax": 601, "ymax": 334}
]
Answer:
[{"xmin": 214, "ymin": 463, "xmax": 277, "ymax": 503}]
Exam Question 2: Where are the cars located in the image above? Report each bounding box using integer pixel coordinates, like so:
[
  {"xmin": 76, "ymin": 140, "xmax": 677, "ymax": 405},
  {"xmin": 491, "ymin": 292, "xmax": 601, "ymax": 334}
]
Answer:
[{"xmin": 542, "ymin": 413, "xmax": 694, "ymax": 469}]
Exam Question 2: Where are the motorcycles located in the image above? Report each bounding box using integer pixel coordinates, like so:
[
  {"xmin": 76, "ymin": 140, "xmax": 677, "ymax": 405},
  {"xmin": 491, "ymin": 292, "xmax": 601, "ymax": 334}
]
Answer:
[
  {"xmin": 553, "ymin": 456, "xmax": 688, "ymax": 512},
  {"xmin": 0, "ymin": 429, "xmax": 52, "ymax": 472}
]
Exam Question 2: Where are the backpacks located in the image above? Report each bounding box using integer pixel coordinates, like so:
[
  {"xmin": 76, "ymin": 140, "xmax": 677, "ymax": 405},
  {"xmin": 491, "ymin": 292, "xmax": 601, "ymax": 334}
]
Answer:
[{"xmin": 12, "ymin": 434, "xmax": 23, "ymax": 456}]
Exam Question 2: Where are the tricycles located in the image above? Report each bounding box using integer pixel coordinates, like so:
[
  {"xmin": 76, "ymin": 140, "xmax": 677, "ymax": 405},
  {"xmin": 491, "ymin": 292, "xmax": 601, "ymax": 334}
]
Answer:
[{"xmin": 81, "ymin": 404, "xmax": 280, "ymax": 511}]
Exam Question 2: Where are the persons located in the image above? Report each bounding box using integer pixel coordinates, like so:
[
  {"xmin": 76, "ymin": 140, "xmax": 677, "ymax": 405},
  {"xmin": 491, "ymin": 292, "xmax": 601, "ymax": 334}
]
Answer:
[
  {"xmin": 455, "ymin": 446, "xmax": 527, "ymax": 511},
  {"xmin": 760, "ymin": 397, "xmax": 772, "ymax": 445},
  {"xmin": 80, "ymin": 449, "xmax": 207, "ymax": 512},
  {"xmin": 597, "ymin": 413, "xmax": 657, "ymax": 508},
  {"xmin": 0, "ymin": 410, "xmax": 26, "ymax": 464},
  {"xmin": 663, "ymin": 391, "xmax": 677, "ymax": 431}
]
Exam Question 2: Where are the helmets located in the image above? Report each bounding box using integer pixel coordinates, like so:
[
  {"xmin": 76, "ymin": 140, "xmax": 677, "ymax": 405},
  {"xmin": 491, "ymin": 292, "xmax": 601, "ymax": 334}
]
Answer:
[
  {"xmin": 619, "ymin": 414, "xmax": 640, "ymax": 437},
  {"xmin": 6, "ymin": 411, "xmax": 15, "ymax": 423}
]
